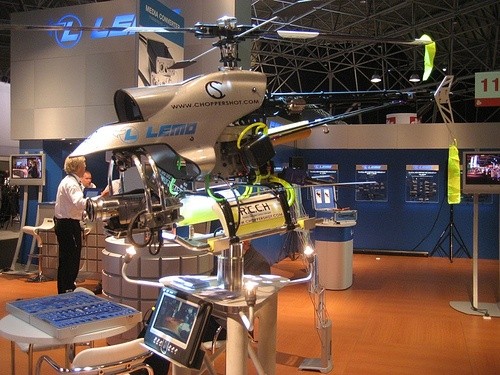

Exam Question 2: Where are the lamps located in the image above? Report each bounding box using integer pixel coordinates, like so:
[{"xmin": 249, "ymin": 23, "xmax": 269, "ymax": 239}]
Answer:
[
  {"xmin": 442, "ymin": 65, "xmax": 448, "ymax": 72},
  {"xmin": 284, "ymin": 246, "xmax": 315, "ymax": 286},
  {"xmin": 120, "ymin": 246, "xmax": 164, "ymax": 288},
  {"xmin": 408, "ymin": 47, "xmax": 421, "ymax": 82},
  {"xmin": 371, "ymin": 46, "xmax": 381, "ymax": 83},
  {"xmin": 239, "ymin": 280, "xmax": 259, "ymax": 332}
]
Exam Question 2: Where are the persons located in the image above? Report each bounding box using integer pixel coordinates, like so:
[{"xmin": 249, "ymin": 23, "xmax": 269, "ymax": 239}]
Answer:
[
  {"xmin": 53, "ymin": 156, "xmax": 110, "ymax": 294},
  {"xmin": 28, "ymin": 159, "xmax": 40, "ymax": 178},
  {"xmin": 81, "ymin": 170, "xmax": 96, "ymax": 193}
]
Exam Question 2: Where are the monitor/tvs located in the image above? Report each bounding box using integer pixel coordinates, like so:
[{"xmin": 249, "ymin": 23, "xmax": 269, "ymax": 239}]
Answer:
[
  {"xmin": 462, "ymin": 151, "xmax": 500, "ymax": 194},
  {"xmin": 9, "ymin": 154, "xmax": 46, "ymax": 185}
]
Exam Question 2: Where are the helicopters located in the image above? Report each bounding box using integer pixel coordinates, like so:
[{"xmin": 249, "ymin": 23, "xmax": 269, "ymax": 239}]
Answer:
[{"xmin": 0, "ymin": 16, "xmax": 459, "ymax": 257}]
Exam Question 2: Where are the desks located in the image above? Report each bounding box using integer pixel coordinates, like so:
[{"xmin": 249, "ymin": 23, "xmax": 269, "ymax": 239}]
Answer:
[{"xmin": 0, "ymin": 303, "xmax": 139, "ymax": 375}]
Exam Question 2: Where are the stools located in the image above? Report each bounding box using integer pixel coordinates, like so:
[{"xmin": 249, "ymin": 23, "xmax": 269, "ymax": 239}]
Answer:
[
  {"xmin": 22, "ymin": 216, "xmax": 55, "ymax": 282},
  {"xmin": 34, "ymin": 337, "xmax": 155, "ymax": 375}
]
